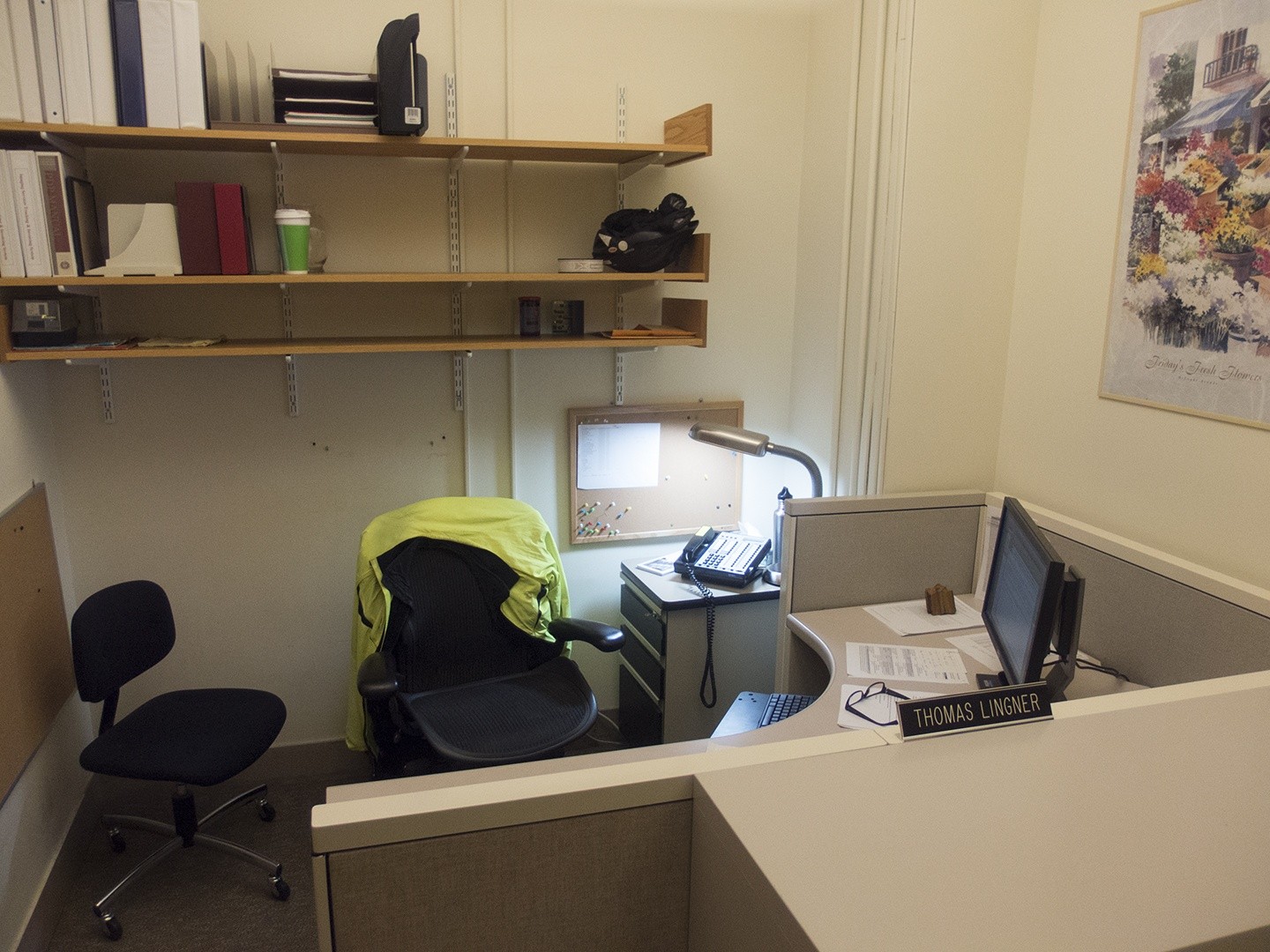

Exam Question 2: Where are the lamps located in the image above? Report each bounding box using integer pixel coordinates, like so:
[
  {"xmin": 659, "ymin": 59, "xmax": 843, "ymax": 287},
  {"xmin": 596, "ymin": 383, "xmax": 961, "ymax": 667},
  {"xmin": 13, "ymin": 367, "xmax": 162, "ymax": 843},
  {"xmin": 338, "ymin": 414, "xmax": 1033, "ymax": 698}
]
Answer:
[{"xmin": 687, "ymin": 422, "xmax": 822, "ymax": 587}]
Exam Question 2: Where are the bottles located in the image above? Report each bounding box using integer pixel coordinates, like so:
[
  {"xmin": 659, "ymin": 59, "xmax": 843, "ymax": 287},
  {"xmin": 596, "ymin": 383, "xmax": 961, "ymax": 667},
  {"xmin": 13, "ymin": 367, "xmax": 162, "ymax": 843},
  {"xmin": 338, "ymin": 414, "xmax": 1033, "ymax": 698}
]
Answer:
[
  {"xmin": 773, "ymin": 486, "xmax": 793, "ymax": 572},
  {"xmin": 519, "ymin": 296, "xmax": 540, "ymax": 336}
]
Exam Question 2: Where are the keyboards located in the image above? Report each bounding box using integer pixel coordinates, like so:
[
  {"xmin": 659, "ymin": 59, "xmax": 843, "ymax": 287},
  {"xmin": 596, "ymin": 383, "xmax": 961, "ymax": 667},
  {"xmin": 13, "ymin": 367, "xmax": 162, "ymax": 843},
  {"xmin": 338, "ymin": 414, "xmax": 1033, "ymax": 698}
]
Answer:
[{"xmin": 756, "ymin": 692, "xmax": 819, "ymax": 730}]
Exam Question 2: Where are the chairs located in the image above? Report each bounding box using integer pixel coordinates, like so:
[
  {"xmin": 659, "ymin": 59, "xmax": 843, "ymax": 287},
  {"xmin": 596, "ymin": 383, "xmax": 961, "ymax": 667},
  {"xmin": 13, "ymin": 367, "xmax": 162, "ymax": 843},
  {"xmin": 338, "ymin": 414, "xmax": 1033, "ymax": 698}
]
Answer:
[
  {"xmin": 357, "ymin": 496, "xmax": 626, "ymax": 779},
  {"xmin": 72, "ymin": 580, "xmax": 291, "ymax": 942}
]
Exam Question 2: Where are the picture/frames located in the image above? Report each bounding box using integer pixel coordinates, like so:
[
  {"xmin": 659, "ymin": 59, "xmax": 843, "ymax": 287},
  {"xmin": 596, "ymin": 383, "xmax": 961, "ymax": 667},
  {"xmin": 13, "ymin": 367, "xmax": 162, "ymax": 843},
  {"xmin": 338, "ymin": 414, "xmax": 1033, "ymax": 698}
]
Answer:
[{"xmin": 1099, "ymin": 0, "xmax": 1270, "ymax": 434}]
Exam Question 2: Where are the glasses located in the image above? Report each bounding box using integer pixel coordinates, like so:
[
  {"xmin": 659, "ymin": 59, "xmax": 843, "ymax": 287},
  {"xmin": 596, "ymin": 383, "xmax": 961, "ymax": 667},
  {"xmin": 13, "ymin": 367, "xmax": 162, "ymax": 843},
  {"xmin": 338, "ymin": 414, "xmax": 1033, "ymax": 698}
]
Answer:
[{"xmin": 845, "ymin": 681, "xmax": 911, "ymax": 726}]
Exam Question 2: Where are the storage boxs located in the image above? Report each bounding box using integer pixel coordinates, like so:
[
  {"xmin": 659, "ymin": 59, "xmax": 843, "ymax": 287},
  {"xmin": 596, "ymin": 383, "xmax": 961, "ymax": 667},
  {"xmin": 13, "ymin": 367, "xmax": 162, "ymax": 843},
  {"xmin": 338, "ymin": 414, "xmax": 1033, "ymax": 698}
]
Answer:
[{"xmin": 175, "ymin": 182, "xmax": 255, "ymax": 275}]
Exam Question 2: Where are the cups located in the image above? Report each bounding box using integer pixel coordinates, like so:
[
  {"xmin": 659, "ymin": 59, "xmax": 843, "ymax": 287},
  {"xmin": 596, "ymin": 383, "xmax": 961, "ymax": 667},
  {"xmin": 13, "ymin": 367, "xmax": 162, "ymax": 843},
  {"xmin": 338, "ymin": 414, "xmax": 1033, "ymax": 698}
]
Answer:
[{"xmin": 274, "ymin": 209, "xmax": 311, "ymax": 276}]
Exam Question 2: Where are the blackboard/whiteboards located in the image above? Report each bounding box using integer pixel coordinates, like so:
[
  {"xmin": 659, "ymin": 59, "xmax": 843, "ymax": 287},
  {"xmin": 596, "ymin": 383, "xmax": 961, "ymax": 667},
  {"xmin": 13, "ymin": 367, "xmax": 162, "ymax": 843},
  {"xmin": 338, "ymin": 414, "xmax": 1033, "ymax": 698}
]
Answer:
[{"xmin": 565, "ymin": 402, "xmax": 746, "ymax": 546}]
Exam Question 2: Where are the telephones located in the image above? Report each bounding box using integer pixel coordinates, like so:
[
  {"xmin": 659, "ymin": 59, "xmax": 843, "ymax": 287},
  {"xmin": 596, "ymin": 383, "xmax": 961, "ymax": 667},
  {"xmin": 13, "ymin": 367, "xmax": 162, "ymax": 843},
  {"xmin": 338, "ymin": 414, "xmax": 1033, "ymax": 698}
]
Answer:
[{"xmin": 673, "ymin": 527, "xmax": 773, "ymax": 589}]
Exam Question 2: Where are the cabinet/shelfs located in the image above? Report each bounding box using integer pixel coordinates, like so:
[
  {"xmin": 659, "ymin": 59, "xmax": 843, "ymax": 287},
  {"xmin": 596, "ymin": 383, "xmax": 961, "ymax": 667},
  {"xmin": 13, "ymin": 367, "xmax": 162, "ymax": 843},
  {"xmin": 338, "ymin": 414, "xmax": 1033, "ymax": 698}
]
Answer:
[
  {"xmin": 308, "ymin": 491, "xmax": 1270, "ymax": 952},
  {"xmin": 0, "ymin": 103, "xmax": 713, "ymax": 364},
  {"xmin": 619, "ymin": 556, "xmax": 780, "ymax": 750}
]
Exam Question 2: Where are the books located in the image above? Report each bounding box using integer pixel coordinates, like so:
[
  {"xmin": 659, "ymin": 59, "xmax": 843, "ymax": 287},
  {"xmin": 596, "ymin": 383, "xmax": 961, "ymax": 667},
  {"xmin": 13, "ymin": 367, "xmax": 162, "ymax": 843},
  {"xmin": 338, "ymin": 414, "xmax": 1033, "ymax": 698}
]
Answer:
[
  {"xmin": 0, "ymin": 147, "xmax": 86, "ymax": 279},
  {"xmin": 272, "ymin": 69, "xmax": 379, "ymax": 128}
]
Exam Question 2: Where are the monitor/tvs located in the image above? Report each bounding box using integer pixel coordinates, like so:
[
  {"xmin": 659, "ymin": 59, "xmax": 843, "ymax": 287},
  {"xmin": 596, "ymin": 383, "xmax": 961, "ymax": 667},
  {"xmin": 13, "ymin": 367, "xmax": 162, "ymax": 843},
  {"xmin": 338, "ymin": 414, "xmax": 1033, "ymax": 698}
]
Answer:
[{"xmin": 975, "ymin": 495, "xmax": 1086, "ymax": 704}]
